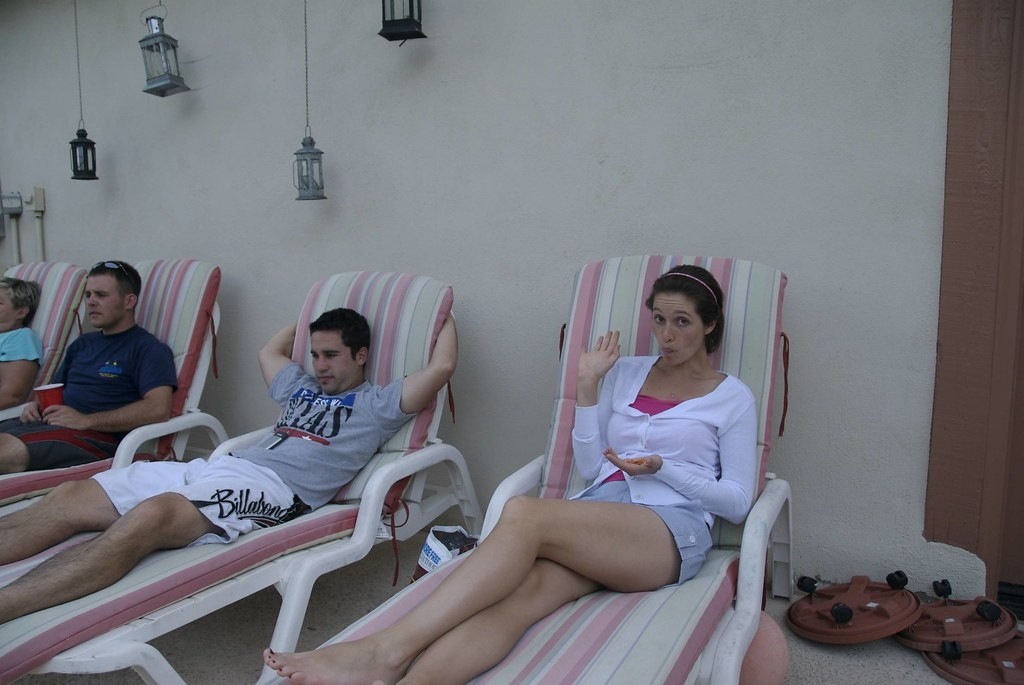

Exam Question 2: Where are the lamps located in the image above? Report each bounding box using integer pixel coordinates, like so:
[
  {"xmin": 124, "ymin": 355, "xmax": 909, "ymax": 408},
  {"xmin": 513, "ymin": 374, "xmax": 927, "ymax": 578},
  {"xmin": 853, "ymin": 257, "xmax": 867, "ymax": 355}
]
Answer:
[
  {"xmin": 377, "ymin": 0, "xmax": 427, "ymax": 47},
  {"xmin": 293, "ymin": 0, "xmax": 327, "ymax": 200},
  {"xmin": 69, "ymin": 1, "xmax": 99, "ymax": 180},
  {"xmin": 138, "ymin": 0, "xmax": 191, "ymax": 97}
]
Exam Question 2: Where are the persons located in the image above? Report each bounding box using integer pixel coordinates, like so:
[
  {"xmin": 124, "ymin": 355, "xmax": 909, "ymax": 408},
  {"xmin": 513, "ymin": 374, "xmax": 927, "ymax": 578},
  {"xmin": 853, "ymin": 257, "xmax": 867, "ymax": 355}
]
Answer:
[
  {"xmin": 0, "ymin": 277, "xmax": 43, "ymax": 411},
  {"xmin": 0, "ymin": 310, "xmax": 457, "ymax": 625},
  {"xmin": 0, "ymin": 261, "xmax": 178, "ymax": 474},
  {"xmin": 264, "ymin": 264, "xmax": 757, "ymax": 684}
]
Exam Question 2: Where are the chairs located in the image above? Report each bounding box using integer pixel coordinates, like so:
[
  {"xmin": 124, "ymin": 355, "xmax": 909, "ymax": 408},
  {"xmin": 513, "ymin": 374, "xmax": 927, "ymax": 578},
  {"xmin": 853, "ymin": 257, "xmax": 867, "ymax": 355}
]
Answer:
[
  {"xmin": 0, "ymin": 269, "xmax": 485, "ymax": 685},
  {"xmin": 0, "ymin": 262, "xmax": 89, "ymax": 423},
  {"xmin": 0, "ymin": 259, "xmax": 222, "ymax": 509},
  {"xmin": 266, "ymin": 253, "xmax": 793, "ymax": 685}
]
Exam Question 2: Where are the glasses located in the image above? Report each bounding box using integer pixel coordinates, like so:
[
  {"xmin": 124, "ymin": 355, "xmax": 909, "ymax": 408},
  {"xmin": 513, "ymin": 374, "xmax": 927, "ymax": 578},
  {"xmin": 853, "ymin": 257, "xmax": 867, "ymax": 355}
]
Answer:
[{"xmin": 92, "ymin": 261, "xmax": 129, "ymax": 278}]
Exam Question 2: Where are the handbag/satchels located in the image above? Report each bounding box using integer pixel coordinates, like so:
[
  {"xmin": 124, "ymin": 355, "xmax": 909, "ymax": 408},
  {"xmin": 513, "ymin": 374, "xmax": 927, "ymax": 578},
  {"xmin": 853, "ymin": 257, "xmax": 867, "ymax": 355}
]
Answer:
[{"xmin": 411, "ymin": 526, "xmax": 480, "ymax": 583}]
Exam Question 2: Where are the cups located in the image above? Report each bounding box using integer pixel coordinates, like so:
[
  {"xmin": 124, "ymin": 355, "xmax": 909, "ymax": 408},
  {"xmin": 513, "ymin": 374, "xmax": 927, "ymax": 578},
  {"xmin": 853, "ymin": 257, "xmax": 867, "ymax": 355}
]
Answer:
[{"xmin": 32, "ymin": 384, "xmax": 64, "ymax": 418}]
